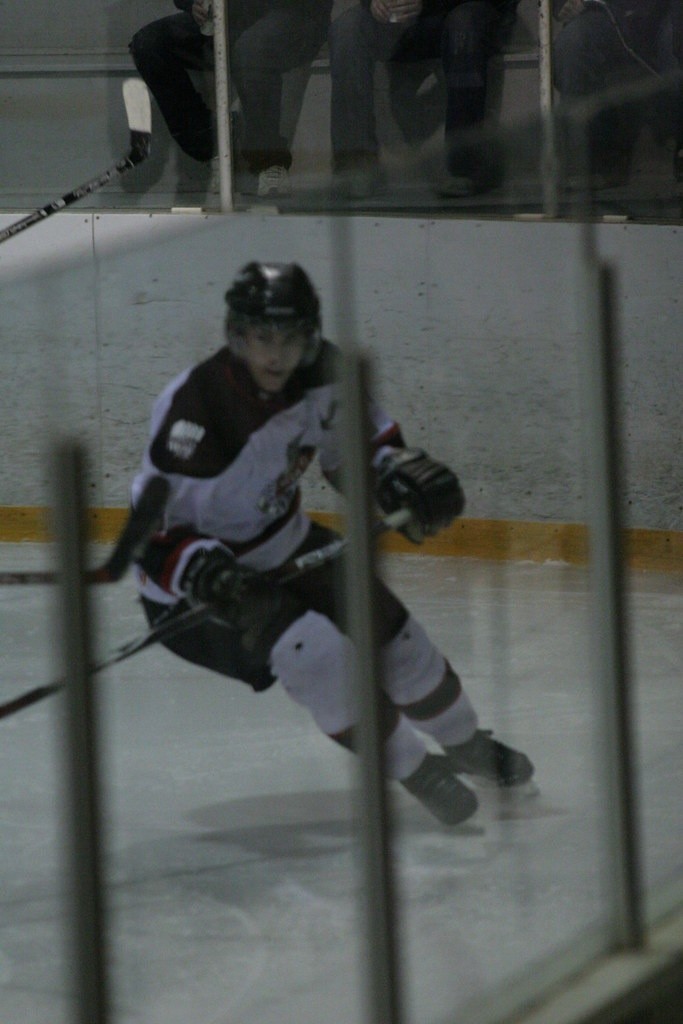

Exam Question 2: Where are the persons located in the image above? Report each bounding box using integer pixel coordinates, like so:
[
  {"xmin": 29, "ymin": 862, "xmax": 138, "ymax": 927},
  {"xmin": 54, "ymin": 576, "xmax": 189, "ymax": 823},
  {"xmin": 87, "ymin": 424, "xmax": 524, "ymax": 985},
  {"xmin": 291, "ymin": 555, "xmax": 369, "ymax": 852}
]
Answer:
[
  {"xmin": 131, "ymin": 264, "xmax": 538, "ymax": 830},
  {"xmin": 129, "ymin": 0, "xmax": 332, "ymax": 196},
  {"xmin": 537, "ymin": 0, "xmax": 683, "ymax": 189},
  {"xmin": 328, "ymin": 0, "xmax": 524, "ymax": 197}
]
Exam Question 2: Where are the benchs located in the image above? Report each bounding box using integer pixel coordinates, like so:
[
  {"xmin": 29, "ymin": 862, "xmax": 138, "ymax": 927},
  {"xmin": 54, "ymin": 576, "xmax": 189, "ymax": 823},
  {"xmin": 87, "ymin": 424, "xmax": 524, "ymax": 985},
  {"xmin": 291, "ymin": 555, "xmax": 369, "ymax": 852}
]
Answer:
[{"xmin": 2, "ymin": 51, "xmax": 672, "ymax": 192}]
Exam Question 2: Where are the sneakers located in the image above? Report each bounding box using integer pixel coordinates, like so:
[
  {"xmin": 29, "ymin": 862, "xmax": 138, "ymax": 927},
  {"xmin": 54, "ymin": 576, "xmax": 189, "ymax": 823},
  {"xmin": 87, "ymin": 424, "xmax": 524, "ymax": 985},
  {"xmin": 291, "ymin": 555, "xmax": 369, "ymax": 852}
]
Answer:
[
  {"xmin": 208, "ymin": 156, "xmax": 222, "ymax": 194},
  {"xmin": 257, "ymin": 164, "xmax": 293, "ymax": 196},
  {"xmin": 398, "ymin": 756, "xmax": 486, "ymax": 834},
  {"xmin": 443, "ymin": 728, "xmax": 540, "ymax": 797}
]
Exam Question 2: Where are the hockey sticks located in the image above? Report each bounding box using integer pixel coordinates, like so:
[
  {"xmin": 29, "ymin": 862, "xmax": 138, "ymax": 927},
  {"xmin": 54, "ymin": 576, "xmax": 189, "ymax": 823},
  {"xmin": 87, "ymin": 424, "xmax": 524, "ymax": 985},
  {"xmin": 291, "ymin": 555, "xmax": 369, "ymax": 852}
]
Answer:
[
  {"xmin": 0, "ymin": 507, "xmax": 416, "ymax": 719},
  {"xmin": 1, "ymin": 77, "xmax": 152, "ymax": 246}
]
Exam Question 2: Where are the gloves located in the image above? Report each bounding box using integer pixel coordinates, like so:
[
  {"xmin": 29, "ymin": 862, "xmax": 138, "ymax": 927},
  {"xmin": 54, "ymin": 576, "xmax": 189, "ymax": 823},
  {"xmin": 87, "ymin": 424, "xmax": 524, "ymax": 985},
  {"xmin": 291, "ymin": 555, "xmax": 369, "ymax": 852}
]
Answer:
[
  {"xmin": 181, "ymin": 549, "xmax": 286, "ymax": 637},
  {"xmin": 373, "ymin": 447, "xmax": 465, "ymax": 546}
]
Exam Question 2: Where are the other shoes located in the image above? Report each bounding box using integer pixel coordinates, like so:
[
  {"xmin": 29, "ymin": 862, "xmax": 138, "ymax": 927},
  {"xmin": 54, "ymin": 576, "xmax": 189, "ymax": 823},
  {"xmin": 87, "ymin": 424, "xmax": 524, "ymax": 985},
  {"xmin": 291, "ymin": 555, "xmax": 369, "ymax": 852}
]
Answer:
[
  {"xmin": 331, "ymin": 167, "xmax": 373, "ymax": 196},
  {"xmin": 565, "ymin": 167, "xmax": 629, "ymax": 190},
  {"xmin": 434, "ymin": 173, "xmax": 494, "ymax": 196}
]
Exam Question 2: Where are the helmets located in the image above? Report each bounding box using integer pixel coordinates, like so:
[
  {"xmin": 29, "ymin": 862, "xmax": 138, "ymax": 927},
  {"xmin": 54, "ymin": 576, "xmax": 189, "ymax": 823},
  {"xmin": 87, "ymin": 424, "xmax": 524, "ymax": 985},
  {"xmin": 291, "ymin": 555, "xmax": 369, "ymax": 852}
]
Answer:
[{"xmin": 223, "ymin": 259, "xmax": 320, "ymax": 322}]
